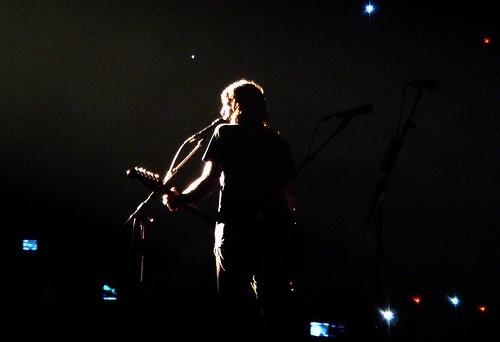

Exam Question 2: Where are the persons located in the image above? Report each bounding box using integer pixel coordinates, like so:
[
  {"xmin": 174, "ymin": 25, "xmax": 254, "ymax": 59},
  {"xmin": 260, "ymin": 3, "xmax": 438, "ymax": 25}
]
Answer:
[{"xmin": 162, "ymin": 79, "xmax": 297, "ymax": 342}]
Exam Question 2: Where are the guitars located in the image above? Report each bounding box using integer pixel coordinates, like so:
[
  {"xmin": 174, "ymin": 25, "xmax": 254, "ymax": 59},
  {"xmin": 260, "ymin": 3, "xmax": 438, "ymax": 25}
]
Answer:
[{"xmin": 125, "ymin": 166, "xmax": 216, "ymax": 227}]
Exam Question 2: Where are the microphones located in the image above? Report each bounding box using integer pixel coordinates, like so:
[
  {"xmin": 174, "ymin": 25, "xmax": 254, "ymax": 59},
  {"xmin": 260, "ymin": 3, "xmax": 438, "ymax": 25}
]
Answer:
[
  {"xmin": 189, "ymin": 117, "xmax": 226, "ymax": 144},
  {"xmin": 408, "ymin": 78, "xmax": 439, "ymax": 90},
  {"xmin": 336, "ymin": 103, "xmax": 374, "ymax": 119}
]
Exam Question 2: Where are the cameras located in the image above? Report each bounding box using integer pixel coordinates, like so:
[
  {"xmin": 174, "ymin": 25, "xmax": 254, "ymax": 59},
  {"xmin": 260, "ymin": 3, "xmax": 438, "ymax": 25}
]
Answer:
[
  {"xmin": 21, "ymin": 238, "xmax": 43, "ymax": 254},
  {"xmin": 307, "ymin": 320, "xmax": 348, "ymax": 339},
  {"xmin": 101, "ymin": 284, "xmax": 119, "ymax": 301}
]
